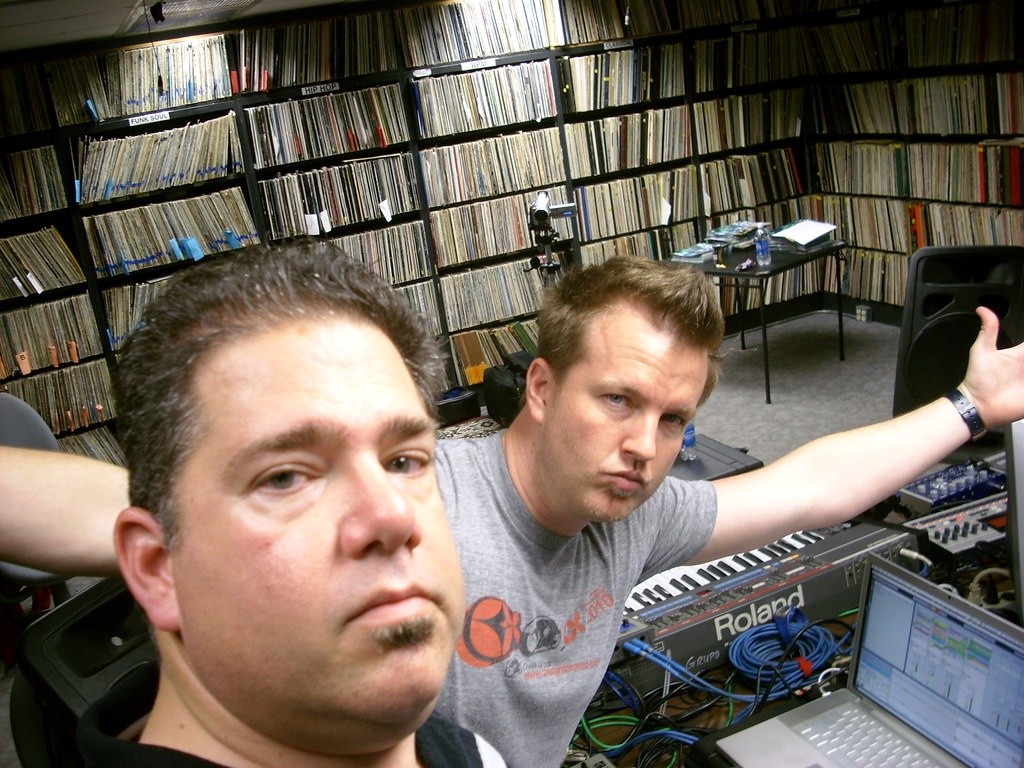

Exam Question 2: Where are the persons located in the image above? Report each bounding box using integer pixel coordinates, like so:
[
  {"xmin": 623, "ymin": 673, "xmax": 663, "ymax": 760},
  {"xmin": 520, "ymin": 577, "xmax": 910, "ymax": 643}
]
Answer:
[
  {"xmin": 0, "ymin": 259, "xmax": 1024, "ymax": 768},
  {"xmin": 76, "ymin": 239, "xmax": 513, "ymax": 768}
]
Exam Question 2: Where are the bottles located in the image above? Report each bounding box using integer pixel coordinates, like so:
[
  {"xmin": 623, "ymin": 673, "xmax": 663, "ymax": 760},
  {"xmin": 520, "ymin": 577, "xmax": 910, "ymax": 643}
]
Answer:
[
  {"xmin": 754, "ymin": 222, "xmax": 772, "ymax": 266},
  {"xmin": 679, "ymin": 418, "xmax": 698, "ymax": 461}
]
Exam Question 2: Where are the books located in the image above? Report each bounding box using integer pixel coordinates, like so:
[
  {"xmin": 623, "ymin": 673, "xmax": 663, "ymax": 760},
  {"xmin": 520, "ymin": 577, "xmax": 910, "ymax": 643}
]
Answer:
[
  {"xmin": 807, "ymin": 0, "xmax": 1023, "ymax": 307},
  {"xmin": 0, "ymin": 0, "xmax": 846, "ymax": 472}
]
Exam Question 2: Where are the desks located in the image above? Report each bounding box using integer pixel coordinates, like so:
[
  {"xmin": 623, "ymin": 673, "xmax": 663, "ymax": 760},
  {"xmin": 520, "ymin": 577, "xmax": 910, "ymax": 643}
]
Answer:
[{"xmin": 661, "ymin": 238, "xmax": 849, "ymax": 404}]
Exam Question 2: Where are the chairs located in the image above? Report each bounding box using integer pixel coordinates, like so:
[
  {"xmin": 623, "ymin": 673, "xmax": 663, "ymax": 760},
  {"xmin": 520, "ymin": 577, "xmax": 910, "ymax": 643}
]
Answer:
[{"xmin": 1, "ymin": 392, "xmax": 78, "ymax": 607}]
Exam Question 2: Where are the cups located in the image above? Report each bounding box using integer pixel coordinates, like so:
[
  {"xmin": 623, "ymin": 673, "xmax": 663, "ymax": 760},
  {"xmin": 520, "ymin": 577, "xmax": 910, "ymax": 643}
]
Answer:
[{"xmin": 712, "ymin": 243, "xmax": 729, "ymax": 265}]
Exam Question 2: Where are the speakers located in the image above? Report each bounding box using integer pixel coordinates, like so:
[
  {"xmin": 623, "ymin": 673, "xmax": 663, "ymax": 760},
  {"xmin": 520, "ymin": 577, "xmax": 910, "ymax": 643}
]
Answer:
[
  {"xmin": 17, "ymin": 575, "xmax": 159, "ymax": 727},
  {"xmin": 891, "ymin": 244, "xmax": 1024, "ymax": 466}
]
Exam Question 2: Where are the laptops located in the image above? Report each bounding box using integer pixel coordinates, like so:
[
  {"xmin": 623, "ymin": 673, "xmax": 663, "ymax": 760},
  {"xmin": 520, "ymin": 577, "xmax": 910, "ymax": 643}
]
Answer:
[{"xmin": 717, "ymin": 552, "xmax": 1024, "ymax": 768}]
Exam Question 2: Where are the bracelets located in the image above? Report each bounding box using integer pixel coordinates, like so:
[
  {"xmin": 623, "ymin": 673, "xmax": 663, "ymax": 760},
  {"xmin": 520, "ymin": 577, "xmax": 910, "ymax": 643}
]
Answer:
[{"xmin": 944, "ymin": 389, "xmax": 988, "ymax": 441}]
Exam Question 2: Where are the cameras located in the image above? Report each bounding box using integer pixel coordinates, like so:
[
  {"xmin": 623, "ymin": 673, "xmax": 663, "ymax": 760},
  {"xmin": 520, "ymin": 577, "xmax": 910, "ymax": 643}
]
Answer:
[{"xmin": 530, "ymin": 190, "xmax": 578, "ymax": 226}]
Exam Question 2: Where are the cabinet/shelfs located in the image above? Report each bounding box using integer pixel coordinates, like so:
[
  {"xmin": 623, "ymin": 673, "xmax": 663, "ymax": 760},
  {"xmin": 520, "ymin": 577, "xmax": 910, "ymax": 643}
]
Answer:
[{"xmin": 1, "ymin": 0, "xmax": 1024, "ymax": 479}]
